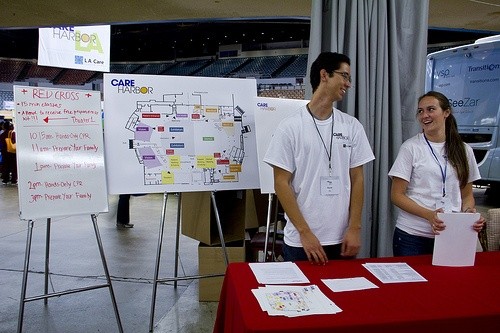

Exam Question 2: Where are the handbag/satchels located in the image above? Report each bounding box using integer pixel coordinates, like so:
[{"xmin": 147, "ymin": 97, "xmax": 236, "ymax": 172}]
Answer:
[{"xmin": 5, "ymin": 130, "xmax": 16, "ymax": 153}]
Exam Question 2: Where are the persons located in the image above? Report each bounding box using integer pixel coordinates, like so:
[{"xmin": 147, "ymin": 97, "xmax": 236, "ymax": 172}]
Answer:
[
  {"xmin": 262, "ymin": 51, "xmax": 375, "ymax": 266},
  {"xmin": 388, "ymin": 91, "xmax": 485, "ymax": 257},
  {"xmin": 117, "ymin": 194, "xmax": 135, "ymax": 230},
  {"xmin": 0, "ymin": 118, "xmax": 17, "ymax": 185}
]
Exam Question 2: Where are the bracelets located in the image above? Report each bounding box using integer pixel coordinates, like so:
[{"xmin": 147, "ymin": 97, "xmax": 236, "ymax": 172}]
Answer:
[{"xmin": 463, "ymin": 207, "xmax": 470, "ymax": 213}]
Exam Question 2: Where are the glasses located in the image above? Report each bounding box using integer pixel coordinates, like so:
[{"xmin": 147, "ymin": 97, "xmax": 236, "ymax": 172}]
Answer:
[{"xmin": 332, "ymin": 71, "xmax": 352, "ymax": 82}]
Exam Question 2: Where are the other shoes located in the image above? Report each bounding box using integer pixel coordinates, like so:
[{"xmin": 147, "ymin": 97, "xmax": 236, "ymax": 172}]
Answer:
[
  {"xmin": 3, "ymin": 180, "xmax": 11, "ymax": 184},
  {"xmin": 117, "ymin": 223, "xmax": 134, "ymax": 228},
  {"xmin": 10, "ymin": 182, "xmax": 18, "ymax": 185}
]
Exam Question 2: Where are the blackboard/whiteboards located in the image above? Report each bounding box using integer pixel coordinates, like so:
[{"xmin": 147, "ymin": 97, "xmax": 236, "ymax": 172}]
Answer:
[{"xmin": 12, "ymin": 85, "xmax": 109, "ymax": 220}]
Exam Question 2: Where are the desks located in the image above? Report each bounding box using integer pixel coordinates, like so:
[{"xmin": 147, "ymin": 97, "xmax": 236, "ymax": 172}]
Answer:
[{"xmin": 213, "ymin": 249, "xmax": 500, "ymax": 333}]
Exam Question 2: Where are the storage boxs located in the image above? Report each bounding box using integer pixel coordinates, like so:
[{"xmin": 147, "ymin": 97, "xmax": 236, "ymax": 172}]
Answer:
[{"xmin": 181, "ymin": 189, "xmax": 259, "ymax": 302}]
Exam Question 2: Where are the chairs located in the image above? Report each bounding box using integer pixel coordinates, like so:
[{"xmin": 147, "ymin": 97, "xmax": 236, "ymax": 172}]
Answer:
[{"xmin": 0, "ymin": 53, "xmax": 309, "ymax": 111}]
